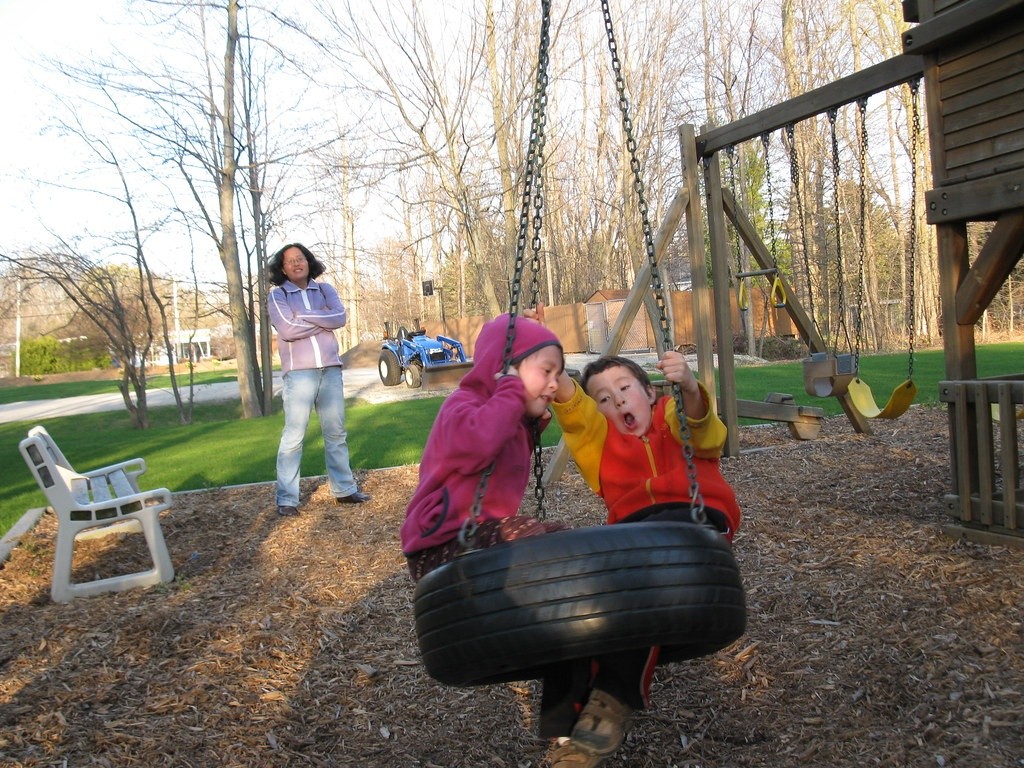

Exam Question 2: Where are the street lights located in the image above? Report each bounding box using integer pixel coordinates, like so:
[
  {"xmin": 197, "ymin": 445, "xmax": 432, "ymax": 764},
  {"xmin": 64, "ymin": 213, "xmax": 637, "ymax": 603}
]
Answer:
[{"xmin": 165, "ymin": 272, "xmax": 182, "ymax": 363}]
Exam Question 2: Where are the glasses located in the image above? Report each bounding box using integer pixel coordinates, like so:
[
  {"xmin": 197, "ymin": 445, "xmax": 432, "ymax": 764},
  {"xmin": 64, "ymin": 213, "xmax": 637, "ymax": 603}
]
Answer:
[{"xmin": 281, "ymin": 254, "xmax": 308, "ymax": 265}]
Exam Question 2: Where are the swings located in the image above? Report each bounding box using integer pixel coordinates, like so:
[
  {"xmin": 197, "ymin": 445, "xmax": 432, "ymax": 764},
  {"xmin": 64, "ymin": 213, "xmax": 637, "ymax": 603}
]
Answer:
[
  {"xmin": 847, "ymin": 93, "xmax": 918, "ymax": 420},
  {"xmin": 410, "ymin": 1, "xmax": 749, "ymax": 686},
  {"xmin": 790, "ymin": 122, "xmax": 860, "ymax": 398}
]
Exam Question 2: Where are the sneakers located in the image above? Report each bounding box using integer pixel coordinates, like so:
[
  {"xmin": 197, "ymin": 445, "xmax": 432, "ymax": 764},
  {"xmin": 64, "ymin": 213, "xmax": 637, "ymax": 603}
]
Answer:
[
  {"xmin": 570, "ymin": 689, "xmax": 633, "ymax": 758},
  {"xmin": 550, "ymin": 740, "xmax": 612, "ymax": 768}
]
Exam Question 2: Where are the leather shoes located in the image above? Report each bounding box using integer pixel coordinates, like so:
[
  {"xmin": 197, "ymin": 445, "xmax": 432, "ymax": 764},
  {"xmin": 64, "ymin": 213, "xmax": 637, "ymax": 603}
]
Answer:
[
  {"xmin": 278, "ymin": 506, "xmax": 300, "ymax": 516},
  {"xmin": 337, "ymin": 492, "xmax": 372, "ymax": 503}
]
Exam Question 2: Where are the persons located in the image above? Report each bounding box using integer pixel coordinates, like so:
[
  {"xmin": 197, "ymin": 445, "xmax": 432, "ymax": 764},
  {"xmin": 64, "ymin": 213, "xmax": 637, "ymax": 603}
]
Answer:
[
  {"xmin": 523, "ymin": 302, "xmax": 741, "ymax": 767},
  {"xmin": 399, "ymin": 313, "xmax": 590, "ymax": 746},
  {"xmin": 266, "ymin": 243, "xmax": 370, "ymax": 516}
]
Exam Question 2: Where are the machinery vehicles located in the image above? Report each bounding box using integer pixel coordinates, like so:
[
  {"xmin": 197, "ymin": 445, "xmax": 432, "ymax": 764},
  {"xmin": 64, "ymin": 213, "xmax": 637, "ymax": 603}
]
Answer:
[{"xmin": 376, "ymin": 318, "xmax": 475, "ymax": 392}]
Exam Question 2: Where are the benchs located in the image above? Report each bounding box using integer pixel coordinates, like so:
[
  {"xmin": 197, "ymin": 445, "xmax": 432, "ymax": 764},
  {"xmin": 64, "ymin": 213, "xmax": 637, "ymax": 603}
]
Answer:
[{"xmin": 18, "ymin": 425, "xmax": 175, "ymax": 604}]
